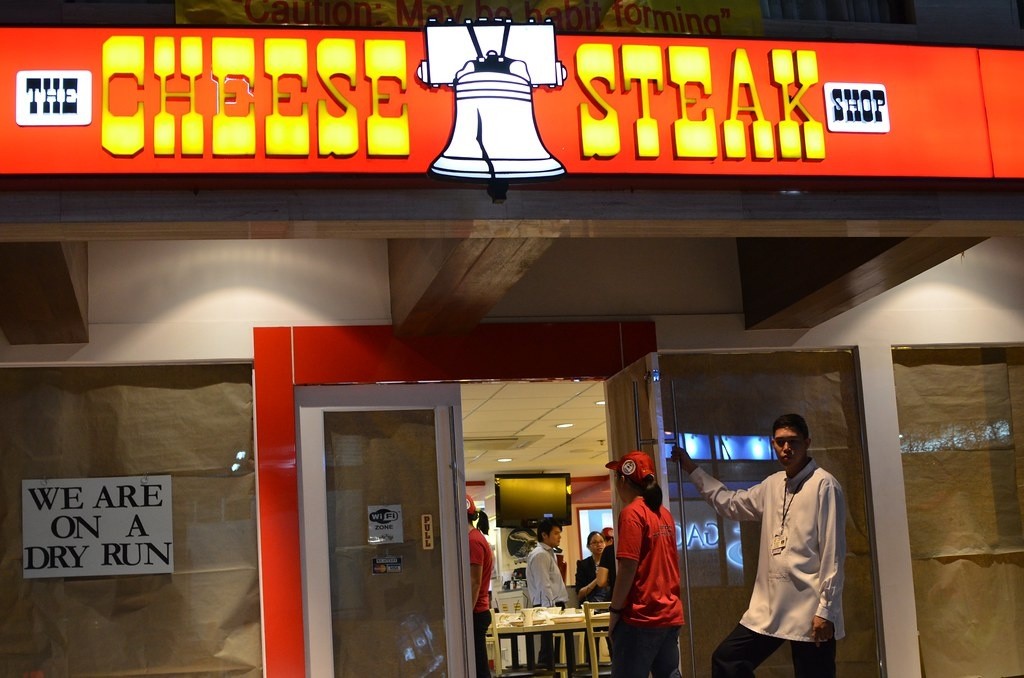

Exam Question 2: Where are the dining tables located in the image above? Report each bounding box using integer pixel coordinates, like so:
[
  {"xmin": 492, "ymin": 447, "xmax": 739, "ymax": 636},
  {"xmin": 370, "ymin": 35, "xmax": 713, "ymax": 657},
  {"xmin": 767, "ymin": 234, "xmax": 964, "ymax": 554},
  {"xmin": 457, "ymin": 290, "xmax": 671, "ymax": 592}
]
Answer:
[{"xmin": 487, "ymin": 618, "xmax": 610, "ymax": 678}]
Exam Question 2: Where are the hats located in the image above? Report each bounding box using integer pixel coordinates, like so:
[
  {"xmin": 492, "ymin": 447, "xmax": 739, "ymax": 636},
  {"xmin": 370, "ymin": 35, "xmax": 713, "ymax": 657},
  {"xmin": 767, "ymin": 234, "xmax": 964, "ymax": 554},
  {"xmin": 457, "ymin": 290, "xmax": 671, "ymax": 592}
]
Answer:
[
  {"xmin": 601, "ymin": 528, "xmax": 614, "ymax": 538},
  {"xmin": 465, "ymin": 493, "xmax": 476, "ymax": 515},
  {"xmin": 605, "ymin": 450, "xmax": 655, "ymax": 487}
]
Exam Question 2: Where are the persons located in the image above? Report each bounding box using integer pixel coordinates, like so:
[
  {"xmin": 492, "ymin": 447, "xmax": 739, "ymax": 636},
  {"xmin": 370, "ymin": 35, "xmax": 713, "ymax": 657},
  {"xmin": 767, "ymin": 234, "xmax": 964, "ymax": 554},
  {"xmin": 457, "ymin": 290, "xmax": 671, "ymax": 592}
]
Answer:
[
  {"xmin": 672, "ymin": 414, "xmax": 846, "ymax": 678},
  {"xmin": 576, "ymin": 531, "xmax": 615, "ymax": 664},
  {"xmin": 466, "ymin": 494, "xmax": 494, "ymax": 678},
  {"xmin": 526, "ymin": 518, "xmax": 569, "ymax": 665},
  {"xmin": 604, "ymin": 450, "xmax": 682, "ymax": 678},
  {"xmin": 595, "ymin": 526, "xmax": 615, "ymax": 599}
]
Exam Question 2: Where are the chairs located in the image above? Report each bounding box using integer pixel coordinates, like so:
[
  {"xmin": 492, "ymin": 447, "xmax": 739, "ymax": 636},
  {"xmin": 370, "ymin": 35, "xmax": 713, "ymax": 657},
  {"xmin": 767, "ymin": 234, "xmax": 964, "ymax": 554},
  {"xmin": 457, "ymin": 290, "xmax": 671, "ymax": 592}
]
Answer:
[
  {"xmin": 485, "ymin": 609, "xmax": 502, "ymax": 677},
  {"xmin": 584, "ymin": 601, "xmax": 611, "ymax": 678}
]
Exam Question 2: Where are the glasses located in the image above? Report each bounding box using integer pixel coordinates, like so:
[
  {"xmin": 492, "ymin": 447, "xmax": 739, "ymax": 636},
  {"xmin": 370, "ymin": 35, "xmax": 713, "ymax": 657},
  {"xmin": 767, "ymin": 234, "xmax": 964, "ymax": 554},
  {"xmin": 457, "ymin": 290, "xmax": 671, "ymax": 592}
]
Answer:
[{"xmin": 589, "ymin": 540, "xmax": 605, "ymax": 546}]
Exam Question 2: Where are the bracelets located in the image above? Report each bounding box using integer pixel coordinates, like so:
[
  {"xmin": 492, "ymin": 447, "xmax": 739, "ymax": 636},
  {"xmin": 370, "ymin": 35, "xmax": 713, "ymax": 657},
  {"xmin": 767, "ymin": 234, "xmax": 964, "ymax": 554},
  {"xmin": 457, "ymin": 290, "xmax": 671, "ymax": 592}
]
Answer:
[{"xmin": 609, "ymin": 604, "xmax": 623, "ymax": 614}]
